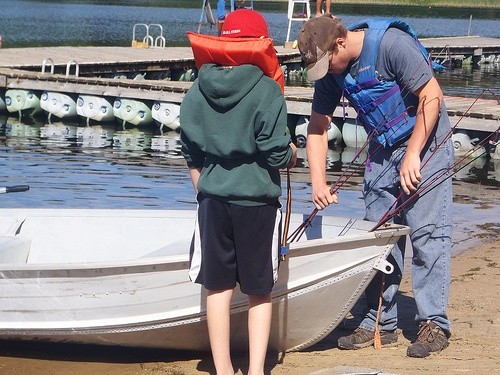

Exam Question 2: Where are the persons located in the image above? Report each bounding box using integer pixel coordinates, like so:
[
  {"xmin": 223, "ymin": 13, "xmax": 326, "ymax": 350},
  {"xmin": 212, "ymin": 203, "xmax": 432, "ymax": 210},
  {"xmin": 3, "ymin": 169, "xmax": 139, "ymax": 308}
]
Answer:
[
  {"xmin": 298, "ymin": 14, "xmax": 455, "ymax": 358},
  {"xmin": 180, "ymin": 9, "xmax": 298, "ymax": 375}
]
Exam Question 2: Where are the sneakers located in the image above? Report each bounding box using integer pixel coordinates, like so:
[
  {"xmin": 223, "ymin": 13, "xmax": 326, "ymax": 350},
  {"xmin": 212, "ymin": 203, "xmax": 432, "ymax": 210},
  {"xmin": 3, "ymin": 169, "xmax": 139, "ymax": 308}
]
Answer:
[
  {"xmin": 407, "ymin": 319, "xmax": 448, "ymax": 358},
  {"xmin": 338, "ymin": 328, "xmax": 399, "ymax": 350}
]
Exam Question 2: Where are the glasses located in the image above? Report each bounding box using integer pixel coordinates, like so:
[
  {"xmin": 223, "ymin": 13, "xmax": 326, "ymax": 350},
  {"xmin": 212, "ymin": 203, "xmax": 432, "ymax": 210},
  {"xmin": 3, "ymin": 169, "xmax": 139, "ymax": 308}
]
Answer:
[{"xmin": 328, "ymin": 41, "xmax": 336, "ymax": 72}]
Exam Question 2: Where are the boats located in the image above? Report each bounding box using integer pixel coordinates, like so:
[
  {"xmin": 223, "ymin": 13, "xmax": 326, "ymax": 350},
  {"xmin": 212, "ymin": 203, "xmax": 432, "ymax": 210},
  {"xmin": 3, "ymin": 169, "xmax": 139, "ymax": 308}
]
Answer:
[{"xmin": 0, "ymin": 183, "xmax": 413, "ymax": 354}]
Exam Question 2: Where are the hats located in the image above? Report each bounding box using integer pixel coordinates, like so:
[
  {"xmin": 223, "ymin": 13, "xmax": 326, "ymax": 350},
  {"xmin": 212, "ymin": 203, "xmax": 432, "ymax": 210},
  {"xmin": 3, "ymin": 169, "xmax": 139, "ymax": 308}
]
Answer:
[
  {"xmin": 221, "ymin": 9, "xmax": 278, "ymax": 53},
  {"xmin": 298, "ymin": 16, "xmax": 341, "ymax": 81}
]
